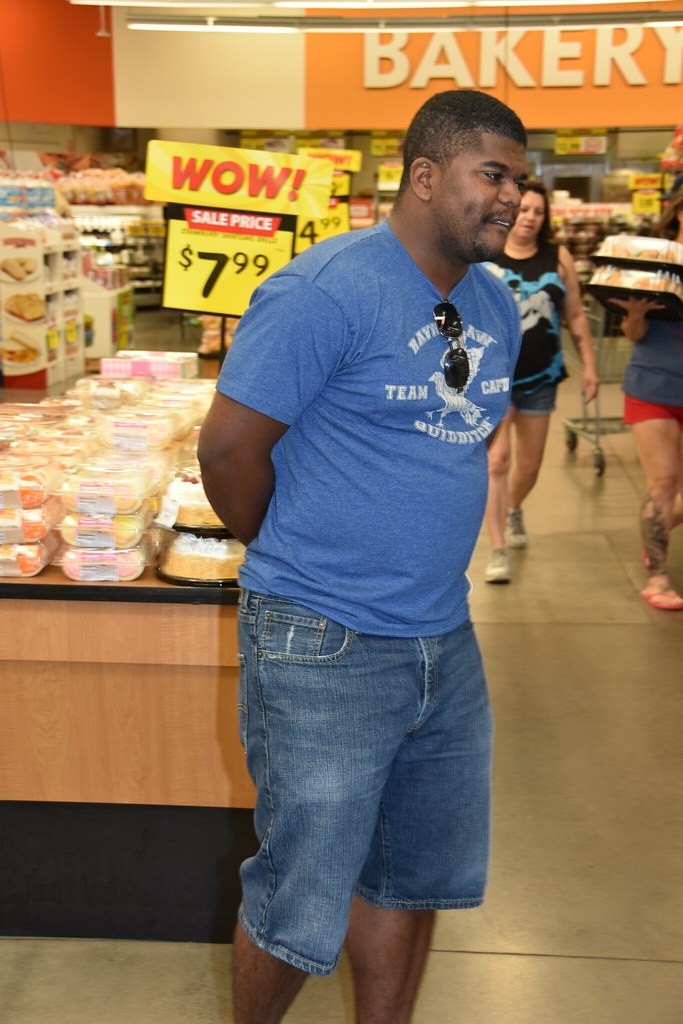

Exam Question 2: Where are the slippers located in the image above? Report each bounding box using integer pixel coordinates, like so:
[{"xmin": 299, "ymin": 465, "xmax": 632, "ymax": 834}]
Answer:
[
  {"xmin": 638, "ymin": 587, "xmax": 683, "ymax": 610},
  {"xmin": 644, "ymin": 547, "xmax": 668, "ymax": 569}
]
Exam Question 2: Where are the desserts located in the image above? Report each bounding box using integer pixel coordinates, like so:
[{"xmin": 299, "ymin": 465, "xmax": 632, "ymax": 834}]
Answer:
[{"xmin": 1, "ymin": 377, "xmax": 218, "ymax": 581}]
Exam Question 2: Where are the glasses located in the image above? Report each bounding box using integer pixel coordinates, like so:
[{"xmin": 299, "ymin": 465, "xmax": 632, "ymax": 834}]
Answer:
[{"xmin": 433, "ymin": 298, "xmax": 470, "ymax": 393}]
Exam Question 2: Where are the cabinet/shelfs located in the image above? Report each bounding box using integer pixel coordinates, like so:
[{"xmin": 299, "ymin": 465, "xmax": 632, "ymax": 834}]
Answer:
[{"xmin": 0, "ymin": 137, "xmax": 683, "ymax": 393}]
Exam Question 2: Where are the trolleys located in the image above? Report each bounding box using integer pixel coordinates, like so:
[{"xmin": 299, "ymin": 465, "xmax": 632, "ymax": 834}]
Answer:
[{"xmin": 562, "ymin": 308, "xmax": 639, "ymax": 473}]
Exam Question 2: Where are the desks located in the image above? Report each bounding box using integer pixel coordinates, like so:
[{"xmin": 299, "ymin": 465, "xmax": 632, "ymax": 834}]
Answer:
[{"xmin": 0, "ymin": 378, "xmax": 279, "ymax": 945}]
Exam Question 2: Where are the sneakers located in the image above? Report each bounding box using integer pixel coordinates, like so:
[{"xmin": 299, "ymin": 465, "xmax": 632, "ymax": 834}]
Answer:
[
  {"xmin": 503, "ymin": 507, "xmax": 527, "ymax": 549},
  {"xmin": 483, "ymin": 544, "xmax": 514, "ymax": 583}
]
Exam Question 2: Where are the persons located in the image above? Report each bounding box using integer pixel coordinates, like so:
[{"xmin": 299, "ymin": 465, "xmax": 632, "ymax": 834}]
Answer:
[
  {"xmin": 195, "ymin": 88, "xmax": 531, "ymax": 1024},
  {"xmin": 610, "ymin": 172, "xmax": 683, "ymax": 611},
  {"xmin": 479, "ymin": 177, "xmax": 599, "ymax": 587}
]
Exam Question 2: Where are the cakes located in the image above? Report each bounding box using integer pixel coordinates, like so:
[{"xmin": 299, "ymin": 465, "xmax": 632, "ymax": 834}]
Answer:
[
  {"xmin": 155, "ymin": 475, "xmax": 230, "ymax": 530},
  {"xmin": 168, "ymin": 532, "xmax": 247, "ymax": 581}
]
